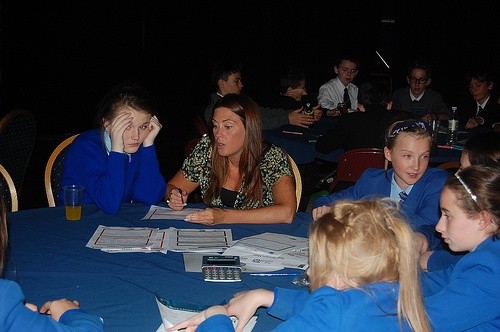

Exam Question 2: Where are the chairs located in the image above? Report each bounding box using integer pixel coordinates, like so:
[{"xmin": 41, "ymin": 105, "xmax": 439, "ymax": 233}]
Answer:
[
  {"xmin": 194, "ymin": 114, "xmax": 208, "ymax": 139},
  {"xmin": 284, "ymin": 153, "xmax": 303, "ymax": 213},
  {"xmin": 0, "ymin": 164, "xmax": 19, "ymax": 214},
  {"xmin": 336, "ymin": 148, "xmax": 391, "ymax": 182},
  {"xmin": 45, "ymin": 134, "xmax": 82, "ymax": 207}
]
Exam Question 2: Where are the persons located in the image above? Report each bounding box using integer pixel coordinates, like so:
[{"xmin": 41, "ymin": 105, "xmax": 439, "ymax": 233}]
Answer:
[
  {"xmin": 59, "ymin": 88, "xmax": 167, "ymax": 214},
  {"xmin": 311, "ymin": 119, "xmax": 448, "ymax": 231},
  {"xmin": 167, "ymin": 194, "xmax": 437, "ymax": 332},
  {"xmin": 414, "ymin": 131, "xmax": 500, "ymax": 272},
  {"xmin": 0, "ymin": 195, "xmax": 105, "ymax": 332},
  {"xmin": 391, "ymin": 67, "xmax": 450, "ymax": 123},
  {"xmin": 317, "ymin": 54, "xmax": 365, "ymax": 116},
  {"xmin": 491, "ymin": 97, "xmax": 500, "ymax": 131},
  {"xmin": 454, "ymin": 70, "xmax": 500, "ymax": 131},
  {"xmin": 204, "ymin": 63, "xmax": 323, "ymax": 130},
  {"xmin": 164, "ymin": 93, "xmax": 297, "ymax": 226},
  {"xmin": 422, "ymin": 165, "xmax": 500, "ymax": 332},
  {"xmin": 259, "ymin": 70, "xmax": 318, "ymax": 110}
]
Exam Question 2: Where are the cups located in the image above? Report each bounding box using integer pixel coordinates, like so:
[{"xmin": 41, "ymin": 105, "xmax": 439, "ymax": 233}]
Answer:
[
  {"xmin": 63, "ymin": 185, "xmax": 85, "ymax": 221},
  {"xmin": 431, "ymin": 119, "xmax": 440, "ymax": 138}
]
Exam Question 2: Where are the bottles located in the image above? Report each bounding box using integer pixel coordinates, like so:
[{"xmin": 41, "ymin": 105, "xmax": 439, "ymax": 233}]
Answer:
[
  {"xmin": 304, "ymin": 103, "xmax": 314, "ymax": 119},
  {"xmin": 447, "ymin": 107, "xmax": 459, "ymax": 146}
]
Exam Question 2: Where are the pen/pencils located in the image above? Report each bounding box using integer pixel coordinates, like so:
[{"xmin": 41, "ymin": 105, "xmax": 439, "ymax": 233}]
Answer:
[{"xmin": 249, "ymin": 271, "xmax": 303, "ymax": 279}]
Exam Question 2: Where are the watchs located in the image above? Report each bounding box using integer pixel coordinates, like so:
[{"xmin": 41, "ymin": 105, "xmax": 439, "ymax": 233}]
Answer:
[{"xmin": 435, "ymin": 112, "xmax": 439, "ymax": 120}]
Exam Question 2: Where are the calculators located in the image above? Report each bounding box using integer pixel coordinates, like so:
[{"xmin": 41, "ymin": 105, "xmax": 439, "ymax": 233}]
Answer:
[{"xmin": 200, "ymin": 252, "xmax": 244, "ymax": 283}]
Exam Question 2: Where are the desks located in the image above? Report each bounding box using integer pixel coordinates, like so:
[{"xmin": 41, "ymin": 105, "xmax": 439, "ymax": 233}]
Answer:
[
  {"xmin": 264, "ymin": 115, "xmax": 475, "ymax": 187},
  {"xmin": 2, "ymin": 203, "xmax": 319, "ymax": 330}
]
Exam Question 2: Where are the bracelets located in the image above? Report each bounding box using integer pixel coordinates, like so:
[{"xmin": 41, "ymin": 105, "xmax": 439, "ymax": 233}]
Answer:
[{"xmin": 479, "ymin": 118, "xmax": 483, "ymax": 123}]
